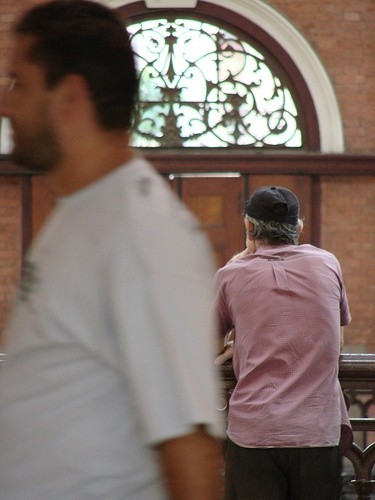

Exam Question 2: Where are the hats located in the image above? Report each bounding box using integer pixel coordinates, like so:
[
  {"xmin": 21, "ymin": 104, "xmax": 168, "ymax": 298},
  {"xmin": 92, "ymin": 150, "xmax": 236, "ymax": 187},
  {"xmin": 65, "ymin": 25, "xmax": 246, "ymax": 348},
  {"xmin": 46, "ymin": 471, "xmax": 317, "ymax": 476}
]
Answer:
[{"xmin": 242, "ymin": 186, "xmax": 299, "ymax": 225}]
